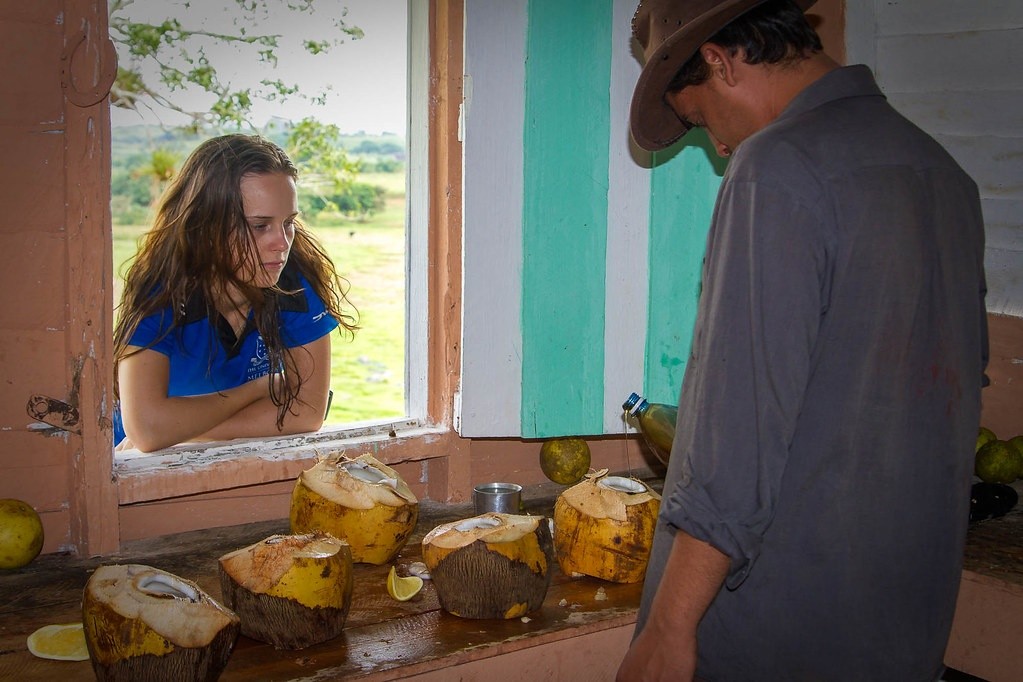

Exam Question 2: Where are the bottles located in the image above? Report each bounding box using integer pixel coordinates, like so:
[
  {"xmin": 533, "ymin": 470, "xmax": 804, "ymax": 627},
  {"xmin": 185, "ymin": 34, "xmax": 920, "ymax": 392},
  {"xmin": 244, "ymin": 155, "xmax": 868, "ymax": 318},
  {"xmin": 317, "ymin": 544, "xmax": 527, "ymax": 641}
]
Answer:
[{"xmin": 622, "ymin": 391, "xmax": 683, "ymax": 468}]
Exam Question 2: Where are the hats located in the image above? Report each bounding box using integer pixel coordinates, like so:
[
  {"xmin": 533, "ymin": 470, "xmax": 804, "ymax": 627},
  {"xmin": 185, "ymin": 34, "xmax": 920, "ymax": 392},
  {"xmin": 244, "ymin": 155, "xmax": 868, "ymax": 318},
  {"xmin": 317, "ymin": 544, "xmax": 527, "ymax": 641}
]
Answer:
[{"xmin": 629, "ymin": 0, "xmax": 817, "ymax": 150}]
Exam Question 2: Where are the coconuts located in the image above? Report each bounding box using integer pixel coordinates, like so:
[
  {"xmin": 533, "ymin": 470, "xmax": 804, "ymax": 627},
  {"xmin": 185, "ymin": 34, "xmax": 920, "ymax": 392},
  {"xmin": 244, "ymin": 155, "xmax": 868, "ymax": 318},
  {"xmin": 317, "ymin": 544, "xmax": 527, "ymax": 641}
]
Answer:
[
  {"xmin": 82, "ymin": 563, "xmax": 243, "ymax": 682},
  {"xmin": 554, "ymin": 468, "xmax": 663, "ymax": 584},
  {"xmin": 220, "ymin": 528, "xmax": 354, "ymax": 651},
  {"xmin": 421, "ymin": 512, "xmax": 555, "ymax": 620},
  {"xmin": 288, "ymin": 448, "xmax": 418, "ymax": 564}
]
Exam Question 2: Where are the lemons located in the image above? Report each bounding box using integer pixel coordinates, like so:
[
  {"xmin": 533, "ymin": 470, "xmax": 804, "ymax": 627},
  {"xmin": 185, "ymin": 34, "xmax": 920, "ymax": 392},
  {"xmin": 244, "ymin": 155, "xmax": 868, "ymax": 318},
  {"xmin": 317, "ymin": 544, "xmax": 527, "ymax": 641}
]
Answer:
[{"xmin": 386, "ymin": 566, "xmax": 424, "ymax": 602}]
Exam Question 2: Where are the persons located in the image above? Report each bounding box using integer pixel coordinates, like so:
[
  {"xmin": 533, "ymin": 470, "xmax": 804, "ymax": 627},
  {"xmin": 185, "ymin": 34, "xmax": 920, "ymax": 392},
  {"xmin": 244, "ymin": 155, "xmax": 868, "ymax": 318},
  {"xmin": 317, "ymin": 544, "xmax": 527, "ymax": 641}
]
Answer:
[
  {"xmin": 608, "ymin": 0, "xmax": 993, "ymax": 682},
  {"xmin": 111, "ymin": 133, "xmax": 362, "ymax": 458}
]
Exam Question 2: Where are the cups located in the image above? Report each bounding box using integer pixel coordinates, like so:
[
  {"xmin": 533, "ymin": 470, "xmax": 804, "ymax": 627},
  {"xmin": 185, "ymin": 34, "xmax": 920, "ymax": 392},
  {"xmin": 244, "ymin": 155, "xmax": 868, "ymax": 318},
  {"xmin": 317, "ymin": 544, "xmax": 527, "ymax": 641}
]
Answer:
[{"xmin": 473, "ymin": 482, "xmax": 524, "ymax": 516}]
